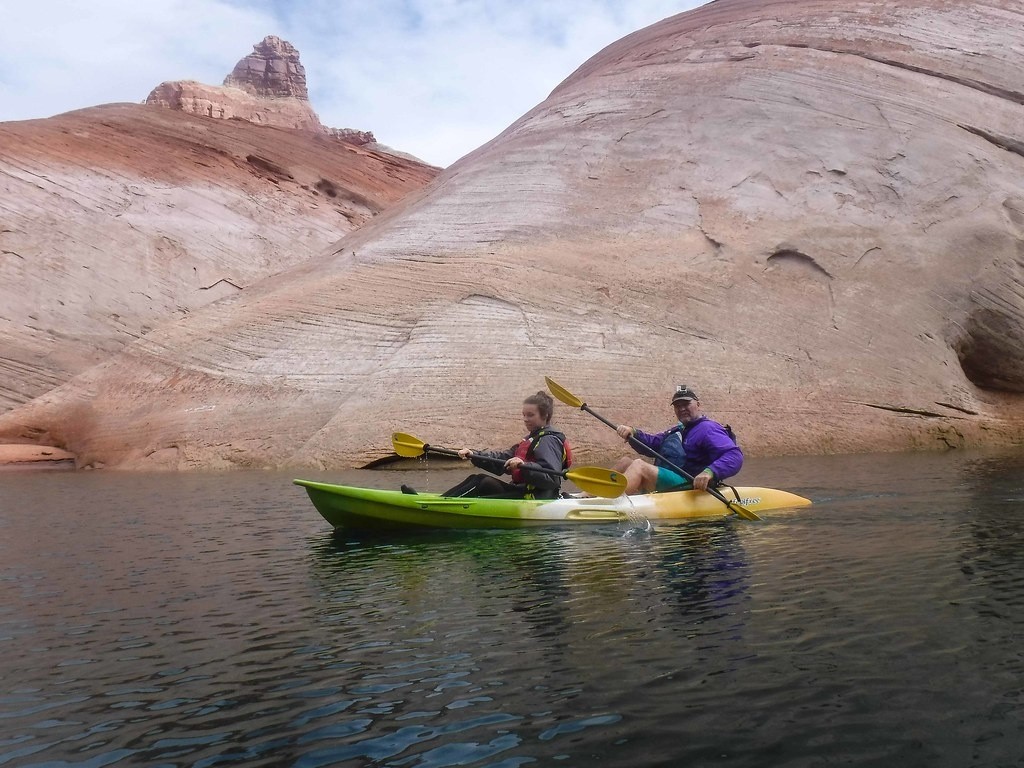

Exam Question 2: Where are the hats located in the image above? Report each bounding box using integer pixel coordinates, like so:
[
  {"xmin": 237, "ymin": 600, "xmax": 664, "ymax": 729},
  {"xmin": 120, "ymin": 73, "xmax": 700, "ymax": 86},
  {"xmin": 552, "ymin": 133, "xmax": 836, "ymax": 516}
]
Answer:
[{"xmin": 670, "ymin": 388, "xmax": 699, "ymax": 406}]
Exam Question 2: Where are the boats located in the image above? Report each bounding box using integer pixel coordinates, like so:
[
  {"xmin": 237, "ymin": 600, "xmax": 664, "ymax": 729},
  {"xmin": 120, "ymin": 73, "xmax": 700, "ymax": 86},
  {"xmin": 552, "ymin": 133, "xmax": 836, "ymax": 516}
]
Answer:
[{"xmin": 292, "ymin": 478, "xmax": 816, "ymax": 534}]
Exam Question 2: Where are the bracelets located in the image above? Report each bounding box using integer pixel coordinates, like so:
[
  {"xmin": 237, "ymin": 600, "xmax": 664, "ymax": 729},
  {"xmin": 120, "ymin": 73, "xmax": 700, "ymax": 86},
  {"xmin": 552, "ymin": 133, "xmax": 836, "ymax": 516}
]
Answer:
[{"xmin": 704, "ymin": 467, "xmax": 715, "ymax": 478}]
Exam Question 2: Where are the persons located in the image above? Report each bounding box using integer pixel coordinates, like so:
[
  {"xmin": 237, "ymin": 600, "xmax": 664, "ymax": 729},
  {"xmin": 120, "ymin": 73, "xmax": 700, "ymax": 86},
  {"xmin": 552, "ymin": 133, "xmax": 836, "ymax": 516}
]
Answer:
[
  {"xmin": 562, "ymin": 385, "xmax": 744, "ymax": 499},
  {"xmin": 401, "ymin": 390, "xmax": 573, "ymax": 499}
]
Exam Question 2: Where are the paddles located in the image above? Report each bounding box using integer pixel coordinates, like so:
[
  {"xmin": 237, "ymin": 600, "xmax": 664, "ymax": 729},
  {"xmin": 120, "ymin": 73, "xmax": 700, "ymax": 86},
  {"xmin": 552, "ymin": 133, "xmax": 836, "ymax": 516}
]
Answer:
[
  {"xmin": 390, "ymin": 432, "xmax": 629, "ymax": 500},
  {"xmin": 543, "ymin": 374, "xmax": 764, "ymax": 523}
]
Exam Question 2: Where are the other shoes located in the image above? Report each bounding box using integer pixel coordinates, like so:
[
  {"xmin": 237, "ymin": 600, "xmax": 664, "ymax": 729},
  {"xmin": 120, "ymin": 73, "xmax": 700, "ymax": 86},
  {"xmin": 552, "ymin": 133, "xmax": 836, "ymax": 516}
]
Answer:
[{"xmin": 401, "ymin": 484, "xmax": 417, "ymax": 494}]
